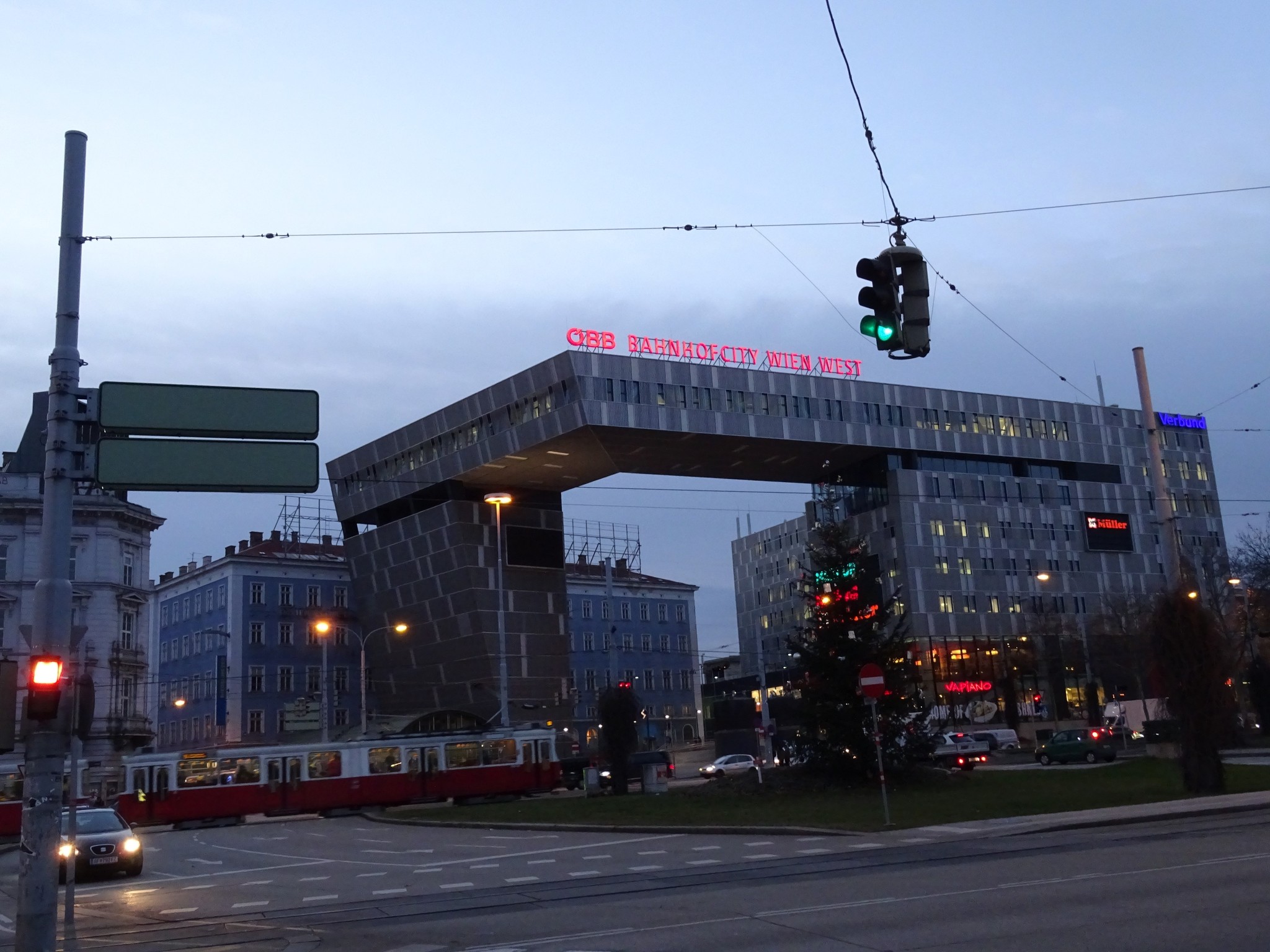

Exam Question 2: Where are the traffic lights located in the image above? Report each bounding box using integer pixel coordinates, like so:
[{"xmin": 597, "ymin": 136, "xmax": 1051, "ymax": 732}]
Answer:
[
  {"xmin": 618, "ymin": 681, "xmax": 631, "ymax": 688},
  {"xmin": 857, "ymin": 244, "xmax": 930, "ymax": 357},
  {"xmin": 28, "ymin": 655, "xmax": 64, "ymax": 686}
]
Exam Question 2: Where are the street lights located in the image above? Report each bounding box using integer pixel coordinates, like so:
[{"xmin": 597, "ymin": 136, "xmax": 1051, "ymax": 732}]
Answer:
[
  {"xmin": 313, "ymin": 620, "xmax": 409, "ymax": 737},
  {"xmin": 1229, "ymin": 578, "xmax": 1256, "ymax": 661},
  {"xmin": 483, "ymin": 491, "xmax": 515, "ymax": 729}
]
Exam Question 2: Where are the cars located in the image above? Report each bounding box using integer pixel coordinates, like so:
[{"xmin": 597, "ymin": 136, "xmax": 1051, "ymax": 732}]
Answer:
[
  {"xmin": 698, "ymin": 754, "xmax": 760, "ymax": 779},
  {"xmin": 773, "ymin": 743, "xmax": 817, "ymax": 766},
  {"xmin": 59, "ymin": 805, "xmax": 144, "ymax": 887},
  {"xmin": 920, "ymin": 729, "xmax": 1020, "ymax": 771},
  {"xmin": 1034, "ymin": 726, "xmax": 1145, "ymax": 765}
]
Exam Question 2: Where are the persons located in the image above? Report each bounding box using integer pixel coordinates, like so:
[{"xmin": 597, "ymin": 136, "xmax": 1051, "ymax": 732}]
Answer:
[
  {"xmin": 408, "ymin": 754, "xmax": 418, "ymax": 769},
  {"xmin": 291, "ymin": 759, "xmax": 300, "ymax": 778},
  {"xmin": 386, "ymin": 749, "xmax": 396, "ymax": 772},
  {"xmin": 316, "ymin": 760, "xmax": 330, "ymax": 777},
  {"xmin": 93, "ymin": 797, "xmax": 105, "ymax": 808},
  {"xmin": 328, "ymin": 752, "xmax": 341, "ymax": 777},
  {"xmin": 236, "ymin": 766, "xmax": 252, "ymax": 783}
]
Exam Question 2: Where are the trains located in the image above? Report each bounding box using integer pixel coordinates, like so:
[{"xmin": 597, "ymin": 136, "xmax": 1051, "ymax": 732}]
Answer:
[{"xmin": 0, "ymin": 728, "xmax": 585, "ymax": 834}]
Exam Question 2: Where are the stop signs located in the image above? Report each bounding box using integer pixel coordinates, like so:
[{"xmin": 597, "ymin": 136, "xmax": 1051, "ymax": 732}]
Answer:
[{"xmin": 859, "ymin": 665, "xmax": 885, "ymax": 698}]
